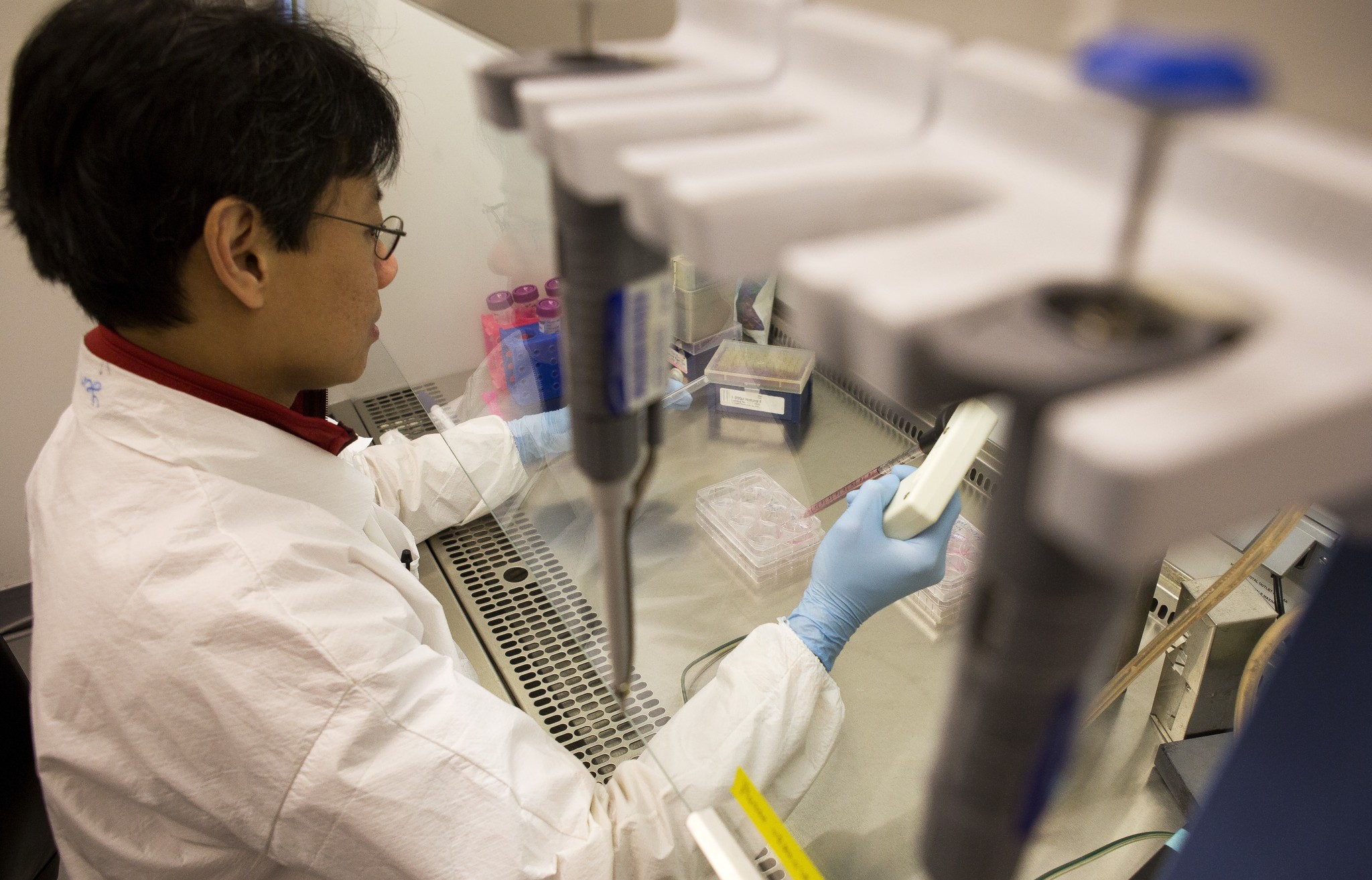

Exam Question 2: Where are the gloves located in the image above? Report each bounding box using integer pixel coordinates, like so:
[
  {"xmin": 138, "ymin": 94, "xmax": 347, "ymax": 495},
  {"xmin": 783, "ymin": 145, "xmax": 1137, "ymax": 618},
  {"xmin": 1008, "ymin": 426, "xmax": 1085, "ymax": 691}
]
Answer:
[
  {"xmin": 509, "ymin": 375, "xmax": 695, "ymax": 470},
  {"xmin": 779, "ymin": 464, "xmax": 961, "ymax": 673}
]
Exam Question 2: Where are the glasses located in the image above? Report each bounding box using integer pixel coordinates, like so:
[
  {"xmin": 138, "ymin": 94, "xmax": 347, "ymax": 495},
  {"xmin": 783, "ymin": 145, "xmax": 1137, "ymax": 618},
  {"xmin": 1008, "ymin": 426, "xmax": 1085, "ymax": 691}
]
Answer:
[{"xmin": 310, "ymin": 197, "xmax": 409, "ymax": 261}]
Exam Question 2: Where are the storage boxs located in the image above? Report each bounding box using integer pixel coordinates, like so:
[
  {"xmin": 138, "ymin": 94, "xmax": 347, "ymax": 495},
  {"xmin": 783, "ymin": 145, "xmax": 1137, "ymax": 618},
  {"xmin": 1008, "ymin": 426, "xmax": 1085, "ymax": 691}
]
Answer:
[
  {"xmin": 665, "ymin": 258, "xmax": 816, "ymax": 425},
  {"xmin": 707, "ymin": 407, "xmax": 812, "ymax": 455}
]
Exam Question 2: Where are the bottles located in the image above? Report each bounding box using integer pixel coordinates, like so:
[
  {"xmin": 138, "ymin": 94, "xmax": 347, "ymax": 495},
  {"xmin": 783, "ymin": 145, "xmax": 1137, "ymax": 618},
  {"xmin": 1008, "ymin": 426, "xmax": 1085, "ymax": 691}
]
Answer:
[
  {"xmin": 545, "ymin": 276, "xmax": 561, "ymax": 297},
  {"xmin": 512, "ymin": 285, "xmax": 539, "ymax": 318},
  {"xmin": 486, "ymin": 291, "xmax": 515, "ymax": 325},
  {"xmin": 536, "ymin": 298, "xmax": 561, "ymax": 333}
]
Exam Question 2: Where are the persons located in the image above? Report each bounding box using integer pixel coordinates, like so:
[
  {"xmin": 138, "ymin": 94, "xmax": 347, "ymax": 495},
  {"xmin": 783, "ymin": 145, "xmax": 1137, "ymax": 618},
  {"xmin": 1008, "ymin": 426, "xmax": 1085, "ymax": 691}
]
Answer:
[{"xmin": 2, "ymin": 0, "xmax": 960, "ymax": 880}]
